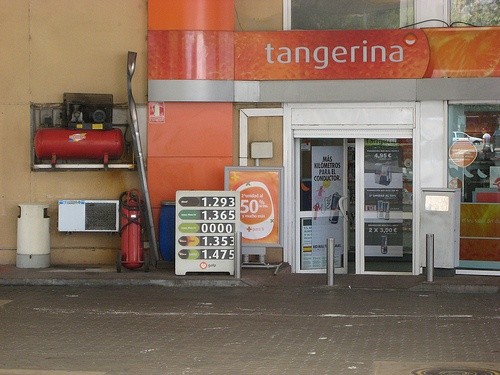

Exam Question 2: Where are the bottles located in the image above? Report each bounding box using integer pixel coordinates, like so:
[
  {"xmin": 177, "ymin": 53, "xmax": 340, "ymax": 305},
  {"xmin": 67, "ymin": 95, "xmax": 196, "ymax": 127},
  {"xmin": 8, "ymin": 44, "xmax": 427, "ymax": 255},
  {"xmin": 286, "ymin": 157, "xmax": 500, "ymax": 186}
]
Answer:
[
  {"xmin": 375, "ymin": 164, "xmax": 382, "ymax": 183},
  {"xmin": 381, "ymin": 235, "xmax": 388, "ymax": 254},
  {"xmin": 379, "ymin": 164, "xmax": 391, "ymax": 186},
  {"xmin": 383, "ymin": 202, "xmax": 390, "ymax": 220},
  {"xmin": 329, "ymin": 192, "xmax": 341, "ymax": 224},
  {"xmin": 377, "ymin": 201, "xmax": 383, "ymax": 219}
]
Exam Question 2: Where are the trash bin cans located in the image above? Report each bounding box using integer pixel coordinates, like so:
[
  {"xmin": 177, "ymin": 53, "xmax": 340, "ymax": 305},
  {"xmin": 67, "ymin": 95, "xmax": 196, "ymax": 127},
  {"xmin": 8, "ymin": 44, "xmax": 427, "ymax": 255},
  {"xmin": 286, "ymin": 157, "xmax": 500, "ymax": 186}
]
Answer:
[{"xmin": 16, "ymin": 204, "xmax": 51, "ymax": 268}]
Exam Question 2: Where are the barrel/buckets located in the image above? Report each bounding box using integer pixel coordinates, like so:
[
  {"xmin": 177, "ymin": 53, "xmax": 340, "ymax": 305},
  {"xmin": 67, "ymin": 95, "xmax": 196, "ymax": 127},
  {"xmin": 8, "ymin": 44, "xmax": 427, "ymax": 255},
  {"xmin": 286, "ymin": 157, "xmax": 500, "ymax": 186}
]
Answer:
[{"xmin": 158, "ymin": 201, "xmax": 175, "ymax": 261}]
